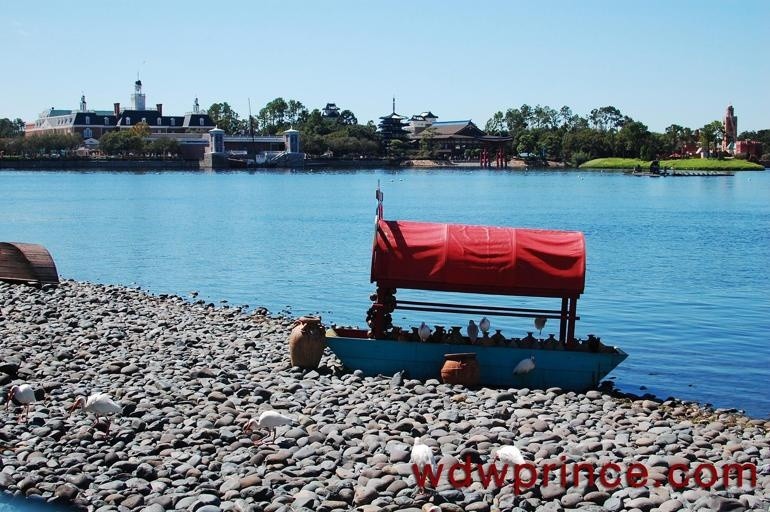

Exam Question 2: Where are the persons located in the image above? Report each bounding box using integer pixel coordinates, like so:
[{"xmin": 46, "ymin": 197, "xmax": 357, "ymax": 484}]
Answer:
[{"xmin": 636, "ymin": 165, "xmax": 642, "ymax": 172}]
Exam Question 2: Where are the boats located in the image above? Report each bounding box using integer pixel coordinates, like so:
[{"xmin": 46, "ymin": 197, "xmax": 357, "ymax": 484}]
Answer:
[{"xmin": 323, "ymin": 180, "xmax": 629, "ymax": 390}]
